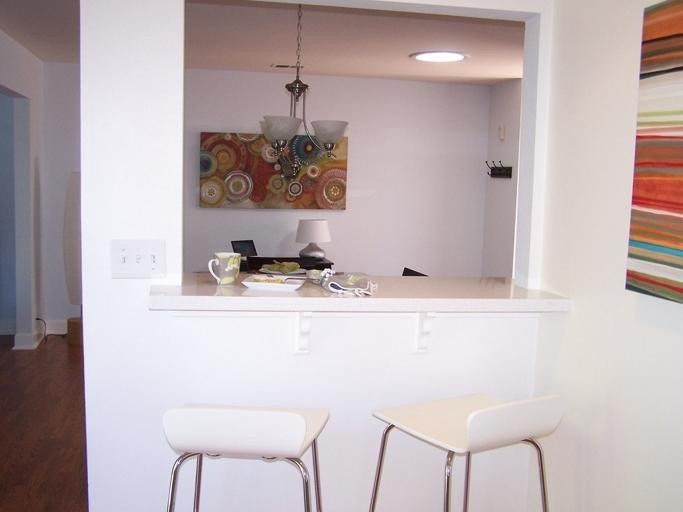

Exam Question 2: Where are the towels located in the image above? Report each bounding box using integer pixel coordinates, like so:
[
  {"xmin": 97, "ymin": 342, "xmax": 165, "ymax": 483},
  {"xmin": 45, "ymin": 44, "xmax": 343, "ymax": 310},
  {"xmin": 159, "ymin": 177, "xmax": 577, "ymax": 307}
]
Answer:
[{"xmin": 321, "ymin": 272, "xmax": 377, "ymax": 297}]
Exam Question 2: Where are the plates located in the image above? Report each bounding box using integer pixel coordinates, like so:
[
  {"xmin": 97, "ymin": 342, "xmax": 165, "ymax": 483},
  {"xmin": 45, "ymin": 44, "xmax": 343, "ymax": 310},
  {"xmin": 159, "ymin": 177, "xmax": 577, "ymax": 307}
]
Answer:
[
  {"xmin": 258, "ymin": 268, "xmax": 307, "ymax": 273},
  {"xmin": 241, "ymin": 275, "xmax": 306, "ymax": 292}
]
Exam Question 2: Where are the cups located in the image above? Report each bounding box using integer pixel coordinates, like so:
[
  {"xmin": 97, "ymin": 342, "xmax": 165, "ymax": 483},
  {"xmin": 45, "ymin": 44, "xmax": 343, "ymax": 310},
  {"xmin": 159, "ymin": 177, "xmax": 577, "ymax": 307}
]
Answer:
[{"xmin": 208, "ymin": 252, "xmax": 241, "ymax": 285}]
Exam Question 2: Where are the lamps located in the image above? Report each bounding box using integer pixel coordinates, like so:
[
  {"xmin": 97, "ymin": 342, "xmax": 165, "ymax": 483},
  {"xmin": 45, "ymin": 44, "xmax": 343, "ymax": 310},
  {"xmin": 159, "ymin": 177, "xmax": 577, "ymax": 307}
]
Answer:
[
  {"xmin": 295, "ymin": 219, "xmax": 331, "ymax": 261},
  {"xmin": 259, "ymin": 4, "xmax": 349, "ymax": 176}
]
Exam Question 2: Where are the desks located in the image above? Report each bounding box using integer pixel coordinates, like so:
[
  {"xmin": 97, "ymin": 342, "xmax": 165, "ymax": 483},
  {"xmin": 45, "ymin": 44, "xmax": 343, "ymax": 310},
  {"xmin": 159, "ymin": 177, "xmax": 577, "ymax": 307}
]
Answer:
[{"xmin": 247, "ymin": 256, "xmax": 334, "ymax": 271}]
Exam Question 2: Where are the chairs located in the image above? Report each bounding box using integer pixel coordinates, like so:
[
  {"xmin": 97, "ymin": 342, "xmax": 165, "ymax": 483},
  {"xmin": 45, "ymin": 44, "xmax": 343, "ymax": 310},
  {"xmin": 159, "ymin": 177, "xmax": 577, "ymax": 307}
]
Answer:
[
  {"xmin": 162, "ymin": 403, "xmax": 330, "ymax": 512},
  {"xmin": 231, "ymin": 240, "xmax": 257, "ymax": 271},
  {"xmin": 369, "ymin": 394, "xmax": 566, "ymax": 512}
]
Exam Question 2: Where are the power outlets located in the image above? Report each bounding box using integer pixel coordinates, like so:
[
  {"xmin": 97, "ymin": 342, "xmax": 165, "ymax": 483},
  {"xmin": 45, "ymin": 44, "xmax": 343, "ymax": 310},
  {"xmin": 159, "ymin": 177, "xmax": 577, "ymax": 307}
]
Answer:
[{"xmin": 110, "ymin": 248, "xmax": 167, "ymax": 274}]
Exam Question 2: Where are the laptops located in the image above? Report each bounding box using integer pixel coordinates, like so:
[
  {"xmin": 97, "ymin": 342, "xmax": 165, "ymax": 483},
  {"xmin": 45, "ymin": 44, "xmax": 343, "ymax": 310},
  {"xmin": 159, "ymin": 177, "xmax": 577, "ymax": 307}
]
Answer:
[{"xmin": 230, "ymin": 240, "xmax": 258, "ymax": 261}]
